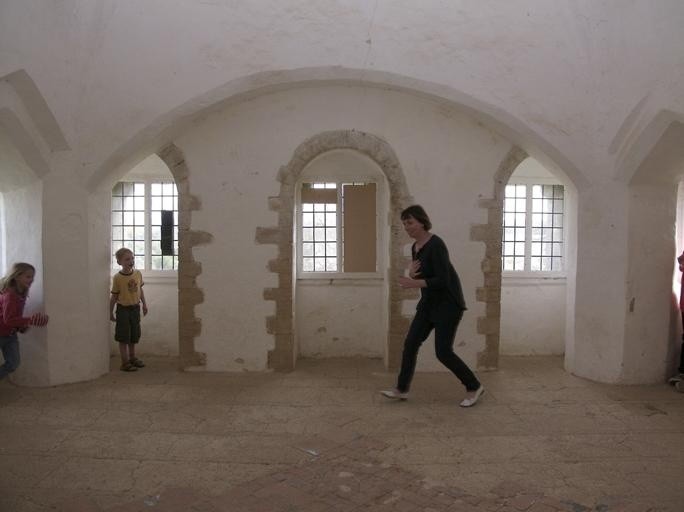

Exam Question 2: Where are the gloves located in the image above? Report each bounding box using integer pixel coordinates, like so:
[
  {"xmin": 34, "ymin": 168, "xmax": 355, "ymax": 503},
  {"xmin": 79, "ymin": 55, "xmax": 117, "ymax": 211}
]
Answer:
[{"xmin": 28, "ymin": 313, "xmax": 49, "ymax": 327}]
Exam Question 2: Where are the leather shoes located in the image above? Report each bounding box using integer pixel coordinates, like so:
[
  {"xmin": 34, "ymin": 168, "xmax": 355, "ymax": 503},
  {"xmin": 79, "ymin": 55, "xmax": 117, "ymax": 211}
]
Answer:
[
  {"xmin": 381, "ymin": 390, "xmax": 409, "ymax": 400},
  {"xmin": 461, "ymin": 386, "xmax": 484, "ymax": 406}
]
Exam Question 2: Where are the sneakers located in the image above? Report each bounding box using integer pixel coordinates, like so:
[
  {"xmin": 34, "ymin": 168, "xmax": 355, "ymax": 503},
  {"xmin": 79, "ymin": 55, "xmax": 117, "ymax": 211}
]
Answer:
[
  {"xmin": 120, "ymin": 357, "xmax": 145, "ymax": 371},
  {"xmin": 669, "ymin": 375, "xmax": 683, "ymax": 392}
]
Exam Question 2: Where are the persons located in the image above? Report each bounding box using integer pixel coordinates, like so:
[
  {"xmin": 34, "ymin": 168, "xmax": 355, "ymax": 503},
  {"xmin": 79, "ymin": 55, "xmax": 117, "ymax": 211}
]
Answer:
[
  {"xmin": 666, "ymin": 248, "xmax": 684, "ymax": 393},
  {"xmin": 377, "ymin": 204, "xmax": 484, "ymax": 408},
  {"xmin": 1, "ymin": 262, "xmax": 50, "ymax": 377},
  {"xmin": 109, "ymin": 247, "xmax": 148, "ymax": 371}
]
